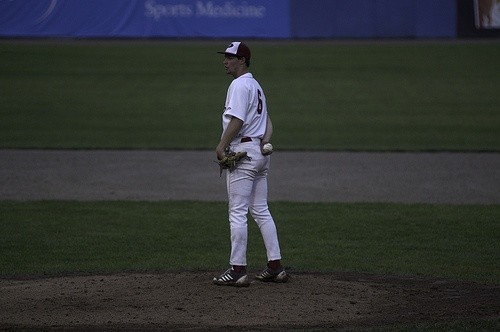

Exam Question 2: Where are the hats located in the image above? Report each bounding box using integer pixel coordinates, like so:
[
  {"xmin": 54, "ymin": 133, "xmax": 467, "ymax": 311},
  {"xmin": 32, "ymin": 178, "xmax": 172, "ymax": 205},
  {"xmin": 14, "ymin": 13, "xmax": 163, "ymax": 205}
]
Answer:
[{"xmin": 217, "ymin": 42, "xmax": 251, "ymax": 60}]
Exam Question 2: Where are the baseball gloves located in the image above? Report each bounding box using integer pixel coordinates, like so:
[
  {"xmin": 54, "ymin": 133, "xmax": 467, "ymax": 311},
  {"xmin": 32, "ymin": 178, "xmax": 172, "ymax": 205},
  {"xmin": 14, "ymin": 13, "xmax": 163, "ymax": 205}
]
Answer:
[{"xmin": 216, "ymin": 151, "xmax": 246, "ymax": 170}]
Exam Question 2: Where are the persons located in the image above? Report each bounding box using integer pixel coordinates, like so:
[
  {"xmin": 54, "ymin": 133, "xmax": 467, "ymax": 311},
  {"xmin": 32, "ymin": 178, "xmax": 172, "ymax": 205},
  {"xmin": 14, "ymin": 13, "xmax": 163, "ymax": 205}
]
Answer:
[{"xmin": 212, "ymin": 42, "xmax": 287, "ymax": 288}]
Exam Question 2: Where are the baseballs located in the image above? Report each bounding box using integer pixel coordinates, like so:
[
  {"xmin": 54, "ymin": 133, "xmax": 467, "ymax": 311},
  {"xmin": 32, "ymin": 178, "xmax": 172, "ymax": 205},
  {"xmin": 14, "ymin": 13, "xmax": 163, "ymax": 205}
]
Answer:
[{"xmin": 262, "ymin": 142, "xmax": 273, "ymax": 155}]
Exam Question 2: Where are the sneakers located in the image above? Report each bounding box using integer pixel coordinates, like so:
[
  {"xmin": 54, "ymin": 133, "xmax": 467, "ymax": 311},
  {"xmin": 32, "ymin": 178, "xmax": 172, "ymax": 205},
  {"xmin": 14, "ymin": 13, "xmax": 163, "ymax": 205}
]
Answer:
[
  {"xmin": 212, "ymin": 264, "xmax": 249, "ymax": 287},
  {"xmin": 254, "ymin": 263, "xmax": 288, "ymax": 283}
]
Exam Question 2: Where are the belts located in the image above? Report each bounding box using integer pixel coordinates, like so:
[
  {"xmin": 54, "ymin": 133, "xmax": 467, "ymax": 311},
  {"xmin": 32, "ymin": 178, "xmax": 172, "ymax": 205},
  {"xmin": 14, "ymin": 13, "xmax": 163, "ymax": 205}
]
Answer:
[{"xmin": 241, "ymin": 137, "xmax": 252, "ymax": 143}]
